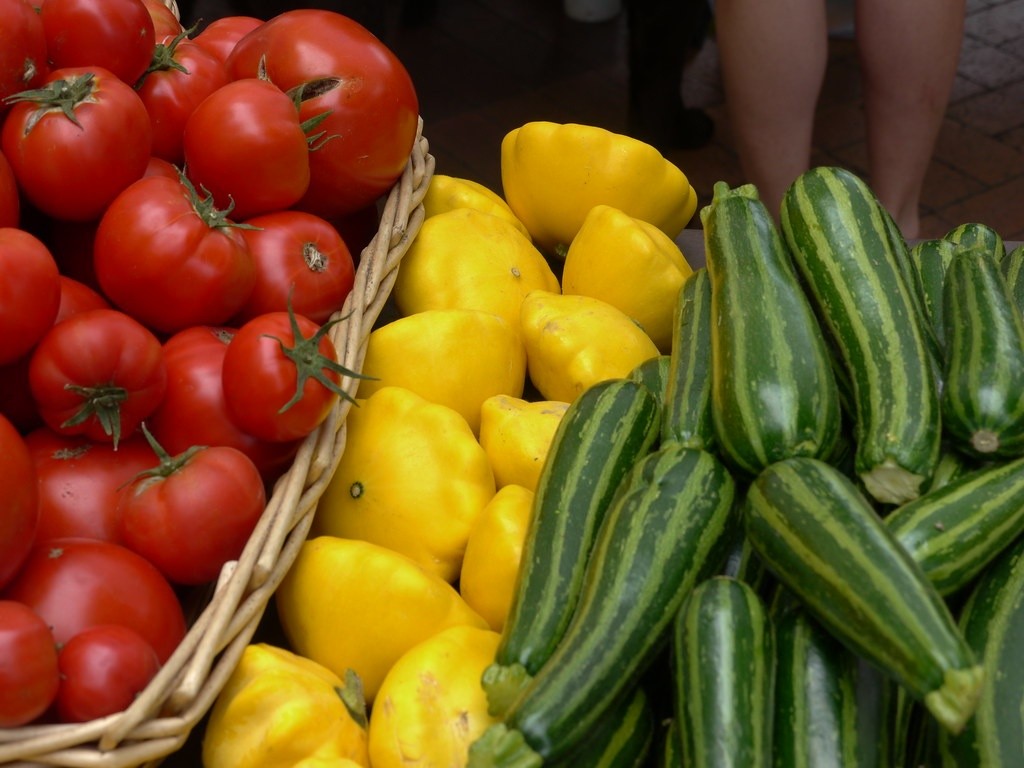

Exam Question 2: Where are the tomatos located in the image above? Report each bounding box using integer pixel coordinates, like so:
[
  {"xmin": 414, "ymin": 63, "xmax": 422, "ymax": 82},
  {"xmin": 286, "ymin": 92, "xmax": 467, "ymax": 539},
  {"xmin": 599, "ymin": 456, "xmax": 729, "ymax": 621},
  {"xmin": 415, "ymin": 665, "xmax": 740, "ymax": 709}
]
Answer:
[{"xmin": 0, "ymin": 0, "xmax": 423, "ymax": 724}]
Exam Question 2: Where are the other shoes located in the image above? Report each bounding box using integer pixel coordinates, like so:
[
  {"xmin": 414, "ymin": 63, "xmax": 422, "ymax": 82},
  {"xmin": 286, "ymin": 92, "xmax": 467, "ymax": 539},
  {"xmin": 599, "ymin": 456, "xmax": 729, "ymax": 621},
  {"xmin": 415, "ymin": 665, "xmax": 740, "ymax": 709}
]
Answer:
[{"xmin": 630, "ymin": 106, "xmax": 713, "ymax": 149}]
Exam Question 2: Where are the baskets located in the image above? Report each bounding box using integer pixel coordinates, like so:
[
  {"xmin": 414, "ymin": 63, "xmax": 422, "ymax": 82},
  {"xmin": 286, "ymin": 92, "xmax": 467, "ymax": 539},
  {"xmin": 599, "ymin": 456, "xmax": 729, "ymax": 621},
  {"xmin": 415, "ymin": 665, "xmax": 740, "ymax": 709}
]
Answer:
[{"xmin": 0, "ymin": 0, "xmax": 437, "ymax": 768}]
